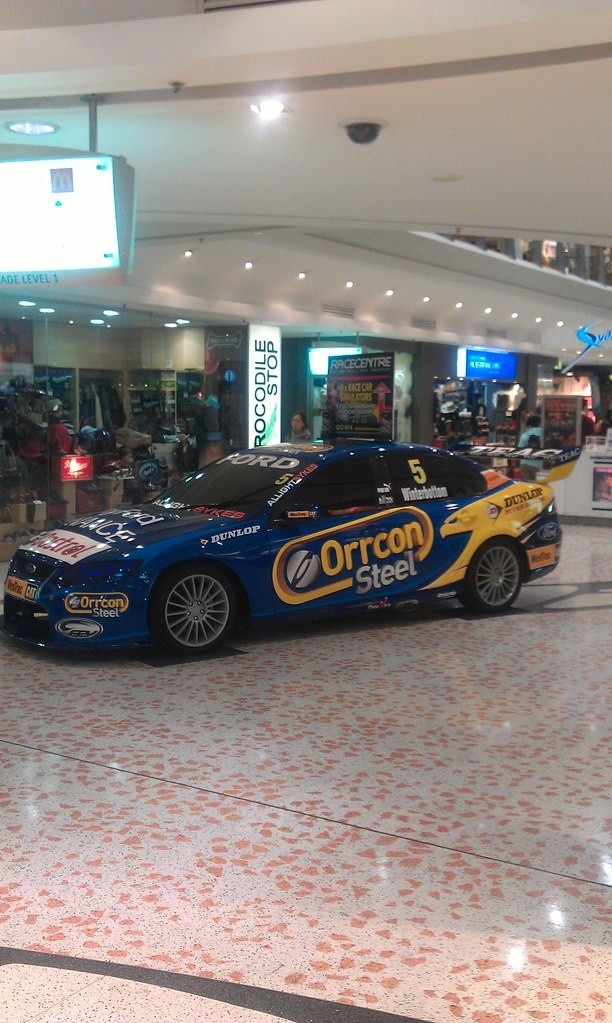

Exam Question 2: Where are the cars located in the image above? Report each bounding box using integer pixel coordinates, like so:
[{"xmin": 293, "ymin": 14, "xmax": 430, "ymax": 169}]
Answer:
[{"xmin": 1, "ymin": 438, "xmax": 564, "ymax": 661}]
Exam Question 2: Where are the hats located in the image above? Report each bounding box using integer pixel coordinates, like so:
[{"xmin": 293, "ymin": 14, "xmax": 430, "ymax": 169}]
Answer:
[
  {"xmin": 439, "ymin": 401, "xmax": 458, "ymax": 414},
  {"xmin": 23, "ymin": 412, "xmax": 48, "ymax": 429},
  {"xmin": 45, "ymin": 398, "xmax": 63, "ymax": 411}
]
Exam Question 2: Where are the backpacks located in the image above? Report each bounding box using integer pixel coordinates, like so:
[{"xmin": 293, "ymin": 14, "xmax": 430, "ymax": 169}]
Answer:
[{"xmin": 526, "ymin": 435, "xmax": 541, "ymax": 449}]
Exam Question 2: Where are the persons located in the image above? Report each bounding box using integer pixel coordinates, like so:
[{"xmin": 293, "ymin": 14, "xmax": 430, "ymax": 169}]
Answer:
[
  {"xmin": 516, "ymin": 414, "xmax": 541, "ymax": 482},
  {"xmin": 42, "ymin": 408, "xmax": 69, "ymax": 460},
  {"xmin": 284, "ymin": 411, "xmax": 314, "ymax": 443}
]
